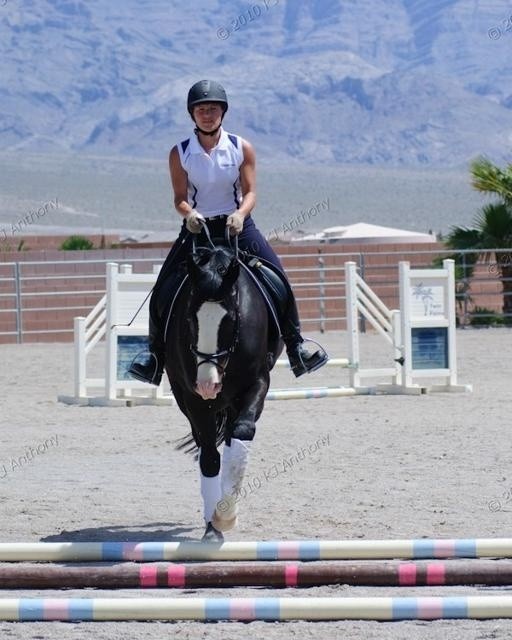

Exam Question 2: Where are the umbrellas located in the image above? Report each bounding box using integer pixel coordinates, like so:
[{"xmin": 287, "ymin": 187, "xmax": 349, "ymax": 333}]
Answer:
[{"xmin": 286, "ymin": 221, "xmax": 441, "ymax": 332}]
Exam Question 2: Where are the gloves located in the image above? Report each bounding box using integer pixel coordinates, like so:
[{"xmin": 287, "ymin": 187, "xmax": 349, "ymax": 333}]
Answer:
[
  {"xmin": 186, "ymin": 209, "xmax": 206, "ymax": 234},
  {"xmin": 226, "ymin": 209, "xmax": 244, "ymax": 236}
]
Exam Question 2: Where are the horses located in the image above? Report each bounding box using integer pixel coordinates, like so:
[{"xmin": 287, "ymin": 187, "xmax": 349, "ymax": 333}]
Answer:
[{"xmin": 159, "ymin": 245, "xmax": 285, "ymax": 542}]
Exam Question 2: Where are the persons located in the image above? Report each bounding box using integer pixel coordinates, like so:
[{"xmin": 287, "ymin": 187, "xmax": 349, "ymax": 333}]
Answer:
[{"xmin": 126, "ymin": 76, "xmax": 330, "ymax": 386}]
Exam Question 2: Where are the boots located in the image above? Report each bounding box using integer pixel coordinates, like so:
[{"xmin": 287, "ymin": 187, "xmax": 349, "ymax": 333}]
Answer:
[
  {"xmin": 286, "ymin": 345, "xmax": 327, "ymax": 379},
  {"xmin": 126, "ymin": 350, "xmax": 165, "ymax": 387}
]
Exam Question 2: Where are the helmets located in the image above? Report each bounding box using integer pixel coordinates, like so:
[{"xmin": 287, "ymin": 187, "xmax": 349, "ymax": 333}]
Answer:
[{"xmin": 187, "ymin": 80, "xmax": 228, "ymax": 116}]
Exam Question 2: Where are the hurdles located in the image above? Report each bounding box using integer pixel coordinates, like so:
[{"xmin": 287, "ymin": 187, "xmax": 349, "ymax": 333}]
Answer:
[{"xmin": 71, "ymin": 259, "xmax": 472, "ymax": 407}]
